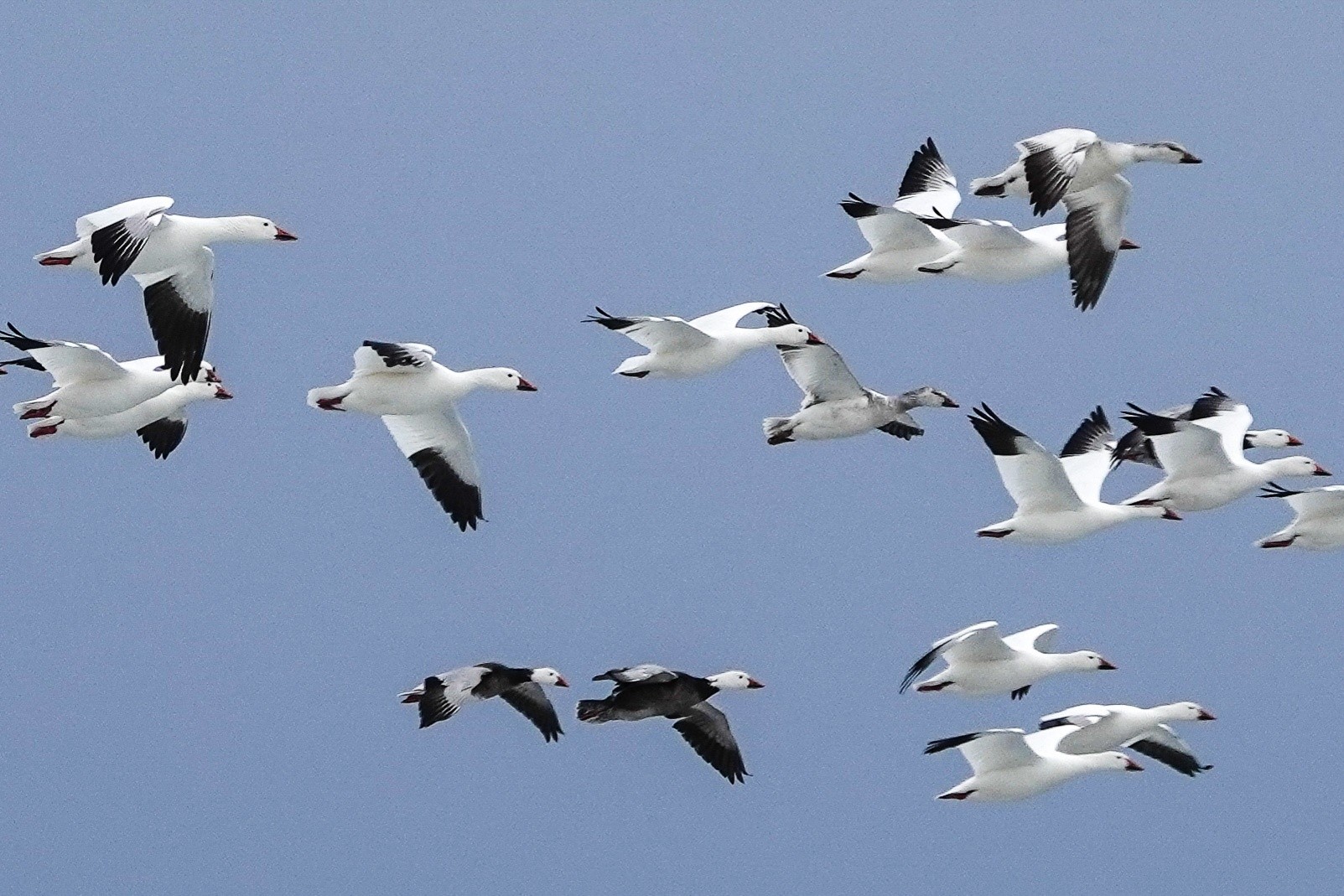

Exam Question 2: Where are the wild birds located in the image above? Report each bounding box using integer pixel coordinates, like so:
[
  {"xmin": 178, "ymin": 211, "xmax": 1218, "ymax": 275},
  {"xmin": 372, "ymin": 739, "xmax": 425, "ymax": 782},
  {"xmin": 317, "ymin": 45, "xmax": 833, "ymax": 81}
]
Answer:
[
  {"xmin": 898, "ymin": 621, "xmax": 1119, "ymax": 701},
  {"xmin": 305, "ymin": 340, "xmax": 539, "ymax": 533},
  {"xmin": 969, "ymin": 127, "xmax": 1204, "ymax": 311},
  {"xmin": 581, "ymin": 302, "xmax": 826, "ymax": 379},
  {"xmin": 764, "ymin": 302, "xmax": 960, "ymax": 448},
  {"xmin": 966, "ymin": 384, "xmax": 1344, "ymax": 556},
  {"xmin": 924, "ymin": 699, "xmax": 1220, "ymax": 803},
  {"xmin": 816, "ymin": 136, "xmax": 1142, "ymax": 284},
  {"xmin": 396, "ymin": 661, "xmax": 766, "ymax": 786},
  {"xmin": 0, "ymin": 322, "xmax": 233, "ymax": 459},
  {"xmin": 32, "ymin": 195, "xmax": 299, "ymax": 386}
]
